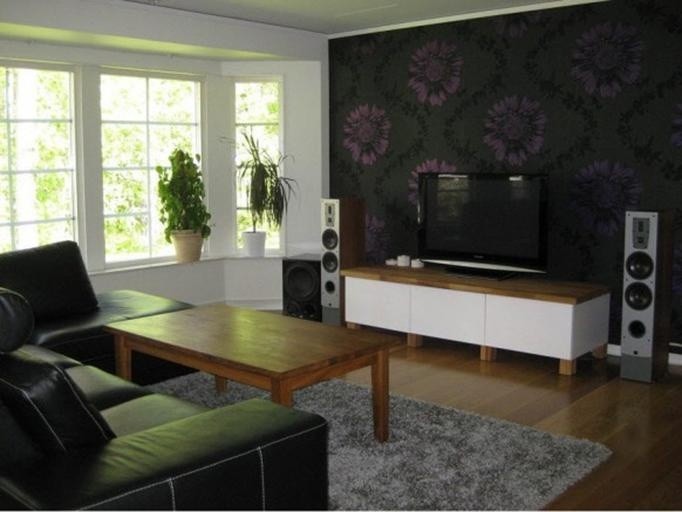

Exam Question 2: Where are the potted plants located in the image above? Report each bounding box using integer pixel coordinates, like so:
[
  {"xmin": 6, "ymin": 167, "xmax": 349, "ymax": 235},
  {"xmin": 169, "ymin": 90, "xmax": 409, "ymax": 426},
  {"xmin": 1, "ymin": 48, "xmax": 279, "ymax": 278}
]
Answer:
[
  {"xmin": 155, "ymin": 148, "xmax": 212, "ymax": 264},
  {"xmin": 233, "ymin": 128, "xmax": 292, "ymax": 257}
]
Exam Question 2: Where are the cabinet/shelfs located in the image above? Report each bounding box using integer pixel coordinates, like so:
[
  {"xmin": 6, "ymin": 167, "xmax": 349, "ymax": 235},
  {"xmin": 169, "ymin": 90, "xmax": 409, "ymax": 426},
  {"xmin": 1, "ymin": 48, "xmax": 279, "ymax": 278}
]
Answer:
[{"xmin": 341, "ymin": 264, "xmax": 612, "ymax": 376}]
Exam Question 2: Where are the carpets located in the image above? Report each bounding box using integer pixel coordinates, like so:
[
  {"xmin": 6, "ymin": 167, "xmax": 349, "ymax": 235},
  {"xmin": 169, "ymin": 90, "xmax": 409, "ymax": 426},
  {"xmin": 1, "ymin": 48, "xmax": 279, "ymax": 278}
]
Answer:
[{"xmin": 137, "ymin": 369, "xmax": 613, "ymax": 511}]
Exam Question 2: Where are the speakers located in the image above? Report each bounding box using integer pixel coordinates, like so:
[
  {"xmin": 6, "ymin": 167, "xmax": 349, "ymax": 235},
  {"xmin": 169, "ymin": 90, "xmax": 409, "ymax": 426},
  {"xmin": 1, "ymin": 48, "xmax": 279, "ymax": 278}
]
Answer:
[
  {"xmin": 320, "ymin": 197, "xmax": 367, "ymax": 327},
  {"xmin": 620, "ymin": 209, "xmax": 674, "ymax": 383},
  {"xmin": 282, "ymin": 253, "xmax": 323, "ymax": 323}
]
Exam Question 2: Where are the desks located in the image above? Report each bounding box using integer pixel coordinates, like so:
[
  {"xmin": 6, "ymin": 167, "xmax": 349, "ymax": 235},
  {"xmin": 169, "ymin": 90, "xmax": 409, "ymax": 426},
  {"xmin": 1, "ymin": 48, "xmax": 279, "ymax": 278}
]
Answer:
[{"xmin": 104, "ymin": 302, "xmax": 405, "ymax": 443}]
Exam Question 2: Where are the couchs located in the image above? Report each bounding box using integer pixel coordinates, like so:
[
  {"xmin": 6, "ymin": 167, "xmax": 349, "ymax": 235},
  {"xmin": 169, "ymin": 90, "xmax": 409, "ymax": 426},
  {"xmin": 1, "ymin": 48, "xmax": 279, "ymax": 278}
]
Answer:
[{"xmin": 0, "ymin": 240, "xmax": 327, "ymax": 511}]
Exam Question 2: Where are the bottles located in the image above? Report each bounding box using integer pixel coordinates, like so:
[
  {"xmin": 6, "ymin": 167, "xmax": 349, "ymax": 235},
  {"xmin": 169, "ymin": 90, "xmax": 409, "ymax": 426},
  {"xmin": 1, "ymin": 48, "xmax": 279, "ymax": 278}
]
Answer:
[{"xmin": 385, "ymin": 255, "xmax": 424, "ymax": 268}]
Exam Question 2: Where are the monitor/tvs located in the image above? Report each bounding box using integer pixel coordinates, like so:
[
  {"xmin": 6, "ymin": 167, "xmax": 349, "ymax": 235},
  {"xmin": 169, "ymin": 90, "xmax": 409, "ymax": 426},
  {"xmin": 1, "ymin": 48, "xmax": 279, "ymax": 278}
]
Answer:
[{"xmin": 417, "ymin": 172, "xmax": 549, "ymax": 281}]
